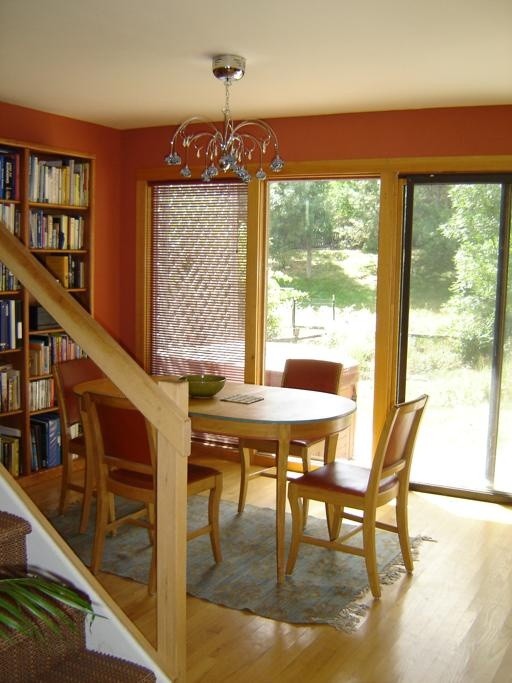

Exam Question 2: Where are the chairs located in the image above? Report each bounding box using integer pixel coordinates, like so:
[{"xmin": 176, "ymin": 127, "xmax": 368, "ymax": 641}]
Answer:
[
  {"xmin": 237, "ymin": 360, "xmax": 342, "ymax": 527},
  {"xmin": 80, "ymin": 389, "xmax": 222, "ymax": 596},
  {"xmin": 54, "ymin": 361, "xmax": 119, "ymax": 537},
  {"xmin": 287, "ymin": 394, "xmax": 427, "ymax": 599}
]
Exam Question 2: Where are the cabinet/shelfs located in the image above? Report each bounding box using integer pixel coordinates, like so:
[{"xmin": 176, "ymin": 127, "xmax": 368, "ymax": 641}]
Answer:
[{"xmin": 0, "ymin": 138, "xmax": 95, "ymax": 522}]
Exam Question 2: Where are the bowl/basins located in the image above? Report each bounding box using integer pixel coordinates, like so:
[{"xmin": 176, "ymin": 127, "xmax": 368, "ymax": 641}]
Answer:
[{"xmin": 179, "ymin": 375, "xmax": 225, "ymax": 398}]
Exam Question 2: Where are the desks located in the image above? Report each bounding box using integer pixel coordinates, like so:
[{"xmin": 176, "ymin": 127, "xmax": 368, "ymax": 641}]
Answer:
[{"xmin": 73, "ymin": 376, "xmax": 355, "ymax": 566}]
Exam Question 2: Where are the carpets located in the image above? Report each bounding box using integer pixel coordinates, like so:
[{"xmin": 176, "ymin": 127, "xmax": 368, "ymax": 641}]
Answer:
[{"xmin": 45, "ymin": 482, "xmax": 437, "ymax": 634}]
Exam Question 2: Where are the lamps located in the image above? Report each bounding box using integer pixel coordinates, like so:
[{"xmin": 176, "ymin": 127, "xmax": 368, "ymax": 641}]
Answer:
[{"xmin": 165, "ymin": 55, "xmax": 285, "ymax": 182}]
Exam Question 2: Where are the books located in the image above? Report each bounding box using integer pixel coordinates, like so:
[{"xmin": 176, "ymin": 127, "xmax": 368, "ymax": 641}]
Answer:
[{"xmin": 0, "ymin": 144, "xmax": 93, "ymax": 479}]
